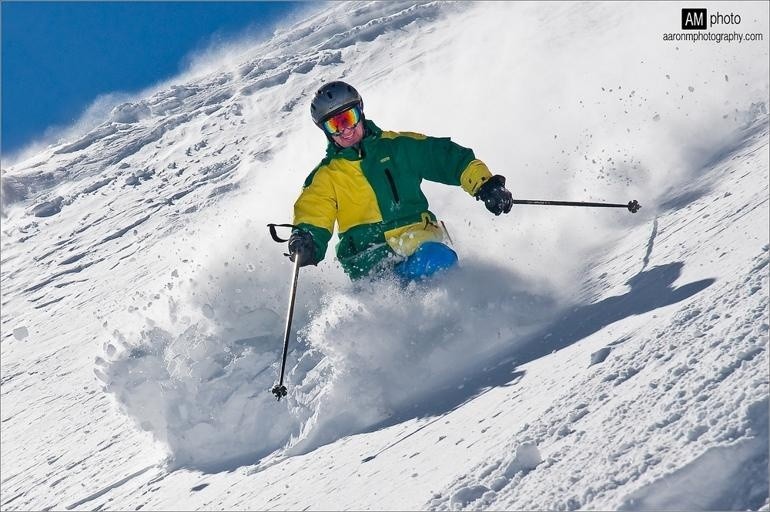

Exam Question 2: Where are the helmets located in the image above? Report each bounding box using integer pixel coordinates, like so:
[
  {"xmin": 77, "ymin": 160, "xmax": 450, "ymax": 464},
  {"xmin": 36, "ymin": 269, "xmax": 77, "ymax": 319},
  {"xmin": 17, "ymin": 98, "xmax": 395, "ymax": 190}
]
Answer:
[{"xmin": 311, "ymin": 81, "xmax": 363, "ymax": 128}]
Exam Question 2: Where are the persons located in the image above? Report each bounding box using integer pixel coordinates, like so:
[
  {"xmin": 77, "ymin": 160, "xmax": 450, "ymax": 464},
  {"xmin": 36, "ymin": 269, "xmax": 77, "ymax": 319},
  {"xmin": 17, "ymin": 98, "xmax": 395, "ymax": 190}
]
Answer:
[{"xmin": 287, "ymin": 81, "xmax": 512, "ymax": 284}]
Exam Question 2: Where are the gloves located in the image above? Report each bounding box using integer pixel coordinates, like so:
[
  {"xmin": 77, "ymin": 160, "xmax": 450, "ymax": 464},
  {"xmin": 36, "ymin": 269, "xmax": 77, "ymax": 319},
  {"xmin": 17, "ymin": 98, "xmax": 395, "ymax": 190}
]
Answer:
[
  {"xmin": 267, "ymin": 223, "xmax": 319, "ymax": 266},
  {"xmin": 474, "ymin": 175, "xmax": 513, "ymax": 216}
]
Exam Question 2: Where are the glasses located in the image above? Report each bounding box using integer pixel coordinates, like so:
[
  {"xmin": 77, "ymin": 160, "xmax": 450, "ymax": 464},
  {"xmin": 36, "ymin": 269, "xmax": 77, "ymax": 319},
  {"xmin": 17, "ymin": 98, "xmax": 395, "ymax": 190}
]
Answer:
[{"xmin": 322, "ymin": 104, "xmax": 362, "ymax": 136}]
